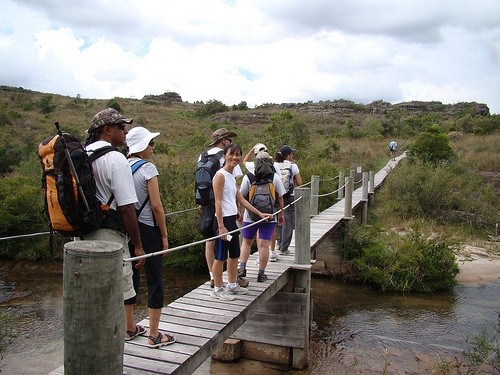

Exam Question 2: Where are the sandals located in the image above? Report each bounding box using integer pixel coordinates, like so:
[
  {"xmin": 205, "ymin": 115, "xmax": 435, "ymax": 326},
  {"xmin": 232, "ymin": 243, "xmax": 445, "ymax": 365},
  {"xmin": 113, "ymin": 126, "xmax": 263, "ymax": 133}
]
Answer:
[
  {"xmin": 124, "ymin": 325, "xmax": 147, "ymax": 340},
  {"xmin": 146, "ymin": 332, "xmax": 176, "ymax": 347}
]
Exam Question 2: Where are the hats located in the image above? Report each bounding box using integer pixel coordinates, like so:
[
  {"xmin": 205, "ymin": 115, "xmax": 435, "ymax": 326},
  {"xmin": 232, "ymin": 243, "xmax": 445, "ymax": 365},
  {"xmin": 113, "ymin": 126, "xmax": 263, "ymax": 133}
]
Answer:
[
  {"xmin": 88, "ymin": 108, "xmax": 134, "ymax": 132},
  {"xmin": 208, "ymin": 128, "xmax": 237, "ymax": 147},
  {"xmin": 280, "ymin": 145, "xmax": 296, "ymax": 154},
  {"xmin": 254, "ymin": 143, "xmax": 267, "ymax": 154},
  {"xmin": 256, "ymin": 152, "xmax": 272, "ymax": 159},
  {"xmin": 125, "ymin": 126, "xmax": 160, "ymax": 156}
]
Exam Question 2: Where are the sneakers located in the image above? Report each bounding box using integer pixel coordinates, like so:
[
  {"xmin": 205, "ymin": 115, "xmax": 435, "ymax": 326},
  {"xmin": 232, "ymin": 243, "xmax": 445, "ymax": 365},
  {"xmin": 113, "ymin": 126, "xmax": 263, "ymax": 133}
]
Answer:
[
  {"xmin": 210, "ymin": 288, "xmax": 234, "ymax": 300},
  {"xmin": 238, "ymin": 269, "xmax": 247, "ymax": 276},
  {"xmin": 257, "ymin": 274, "xmax": 267, "ymax": 282},
  {"xmin": 226, "ymin": 283, "xmax": 248, "ymax": 294}
]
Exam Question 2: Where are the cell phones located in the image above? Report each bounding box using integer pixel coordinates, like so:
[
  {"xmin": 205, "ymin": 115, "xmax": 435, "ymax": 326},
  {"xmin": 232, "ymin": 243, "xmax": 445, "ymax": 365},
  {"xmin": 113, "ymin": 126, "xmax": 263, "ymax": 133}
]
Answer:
[{"xmin": 227, "ymin": 235, "xmax": 232, "ymax": 241}]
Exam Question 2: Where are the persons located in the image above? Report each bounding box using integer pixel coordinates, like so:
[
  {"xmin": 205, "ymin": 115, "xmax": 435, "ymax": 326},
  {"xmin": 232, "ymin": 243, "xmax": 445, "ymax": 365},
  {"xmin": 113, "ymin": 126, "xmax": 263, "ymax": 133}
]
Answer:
[
  {"xmin": 389, "ymin": 140, "xmax": 398, "ymax": 161},
  {"xmin": 85, "ymin": 109, "xmax": 146, "ymax": 298},
  {"xmin": 274, "ymin": 146, "xmax": 302, "ymax": 256},
  {"xmin": 242, "ymin": 142, "xmax": 282, "ymax": 266},
  {"xmin": 125, "ymin": 126, "xmax": 176, "ymax": 347},
  {"xmin": 210, "ymin": 145, "xmax": 272, "ymax": 301},
  {"xmin": 237, "ymin": 152, "xmax": 286, "ymax": 282},
  {"xmin": 198, "ymin": 128, "xmax": 249, "ymax": 287}
]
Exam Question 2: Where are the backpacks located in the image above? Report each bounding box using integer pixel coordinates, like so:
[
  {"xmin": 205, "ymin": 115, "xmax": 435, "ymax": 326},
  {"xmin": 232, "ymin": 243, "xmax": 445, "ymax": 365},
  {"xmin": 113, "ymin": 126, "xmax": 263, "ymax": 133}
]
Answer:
[
  {"xmin": 248, "ymin": 179, "xmax": 275, "ymax": 222},
  {"xmin": 39, "ymin": 132, "xmax": 127, "ymax": 237},
  {"xmin": 197, "ymin": 200, "xmax": 219, "ymax": 238},
  {"xmin": 275, "ymin": 162, "xmax": 294, "ymax": 194},
  {"xmin": 193, "ymin": 150, "xmax": 225, "ymax": 206}
]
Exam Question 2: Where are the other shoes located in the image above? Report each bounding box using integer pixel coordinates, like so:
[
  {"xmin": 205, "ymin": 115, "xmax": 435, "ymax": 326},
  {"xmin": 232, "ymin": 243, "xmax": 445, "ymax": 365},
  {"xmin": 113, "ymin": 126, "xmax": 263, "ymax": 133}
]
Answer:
[
  {"xmin": 256, "ymin": 260, "xmax": 260, "ymax": 266},
  {"xmin": 277, "ymin": 245, "xmax": 281, "ymax": 249},
  {"xmin": 237, "ymin": 277, "xmax": 249, "ymax": 287},
  {"xmin": 270, "ymin": 254, "xmax": 279, "ymax": 261},
  {"xmin": 211, "ymin": 280, "xmax": 215, "ymax": 288},
  {"xmin": 279, "ymin": 249, "xmax": 291, "ymax": 254}
]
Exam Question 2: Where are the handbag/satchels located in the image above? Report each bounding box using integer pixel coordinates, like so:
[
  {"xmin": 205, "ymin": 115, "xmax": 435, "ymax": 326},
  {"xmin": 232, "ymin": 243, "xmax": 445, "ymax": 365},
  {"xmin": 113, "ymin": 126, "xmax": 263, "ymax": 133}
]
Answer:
[{"xmin": 389, "ymin": 146, "xmax": 393, "ymax": 151}]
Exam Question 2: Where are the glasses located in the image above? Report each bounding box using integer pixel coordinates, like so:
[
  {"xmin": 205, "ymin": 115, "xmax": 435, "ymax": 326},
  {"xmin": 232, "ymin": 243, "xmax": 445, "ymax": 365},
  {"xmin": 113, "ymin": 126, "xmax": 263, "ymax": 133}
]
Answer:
[
  {"xmin": 224, "ymin": 137, "xmax": 232, "ymax": 144},
  {"xmin": 109, "ymin": 124, "xmax": 125, "ymax": 130},
  {"xmin": 150, "ymin": 142, "xmax": 154, "ymax": 147}
]
